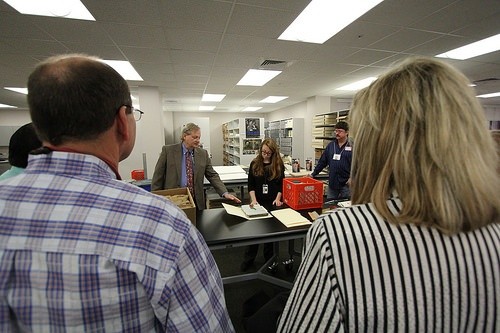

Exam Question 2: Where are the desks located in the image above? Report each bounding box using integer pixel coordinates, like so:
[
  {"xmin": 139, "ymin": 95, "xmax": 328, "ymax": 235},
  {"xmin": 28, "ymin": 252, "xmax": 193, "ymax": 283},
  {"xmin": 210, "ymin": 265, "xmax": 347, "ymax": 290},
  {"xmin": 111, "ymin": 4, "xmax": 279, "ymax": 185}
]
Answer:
[{"xmin": 128, "ymin": 168, "xmax": 351, "ymax": 292}]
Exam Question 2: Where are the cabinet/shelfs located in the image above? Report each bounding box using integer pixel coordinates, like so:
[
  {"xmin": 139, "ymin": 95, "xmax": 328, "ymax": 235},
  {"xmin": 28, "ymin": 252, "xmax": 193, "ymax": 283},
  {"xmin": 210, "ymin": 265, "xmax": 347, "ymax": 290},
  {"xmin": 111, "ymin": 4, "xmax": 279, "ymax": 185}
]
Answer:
[{"xmin": 181, "ymin": 108, "xmax": 350, "ymax": 167}]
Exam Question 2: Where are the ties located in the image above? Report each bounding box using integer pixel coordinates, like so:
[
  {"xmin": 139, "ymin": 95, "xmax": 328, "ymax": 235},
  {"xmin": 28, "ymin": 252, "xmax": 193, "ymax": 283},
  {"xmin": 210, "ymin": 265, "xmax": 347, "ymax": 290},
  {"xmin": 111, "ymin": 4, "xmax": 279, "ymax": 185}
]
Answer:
[{"xmin": 185, "ymin": 151, "xmax": 194, "ymax": 197}]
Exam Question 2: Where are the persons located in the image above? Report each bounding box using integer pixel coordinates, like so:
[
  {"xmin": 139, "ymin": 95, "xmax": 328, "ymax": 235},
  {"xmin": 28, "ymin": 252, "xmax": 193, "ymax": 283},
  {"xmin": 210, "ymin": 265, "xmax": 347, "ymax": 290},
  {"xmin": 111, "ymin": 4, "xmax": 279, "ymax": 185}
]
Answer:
[
  {"xmin": 151, "ymin": 123, "xmax": 240, "ymax": 210},
  {"xmin": 243, "ymin": 119, "xmax": 260, "ymax": 151},
  {"xmin": 0, "ymin": 122, "xmax": 42, "ymax": 182},
  {"xmin": 0, "ymin": 54, "xmax": 236, "ymax": 333},
  {"xmin": 240, "ymin": 138, "xmax": 286, "ymax": 271},
  {"xmin": 308, "ymin": 121, "xmax": 351, "ymax": 199},
  {"xmin": 275, "ymin": 57, "xmax": 500, "ymax": 333}
]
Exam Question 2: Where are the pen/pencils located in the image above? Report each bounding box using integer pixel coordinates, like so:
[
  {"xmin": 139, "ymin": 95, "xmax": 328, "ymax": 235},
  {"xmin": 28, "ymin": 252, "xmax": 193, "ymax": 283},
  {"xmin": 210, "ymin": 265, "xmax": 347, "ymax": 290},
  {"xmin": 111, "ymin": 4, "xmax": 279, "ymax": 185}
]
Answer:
[{"xmin": 250, "ymin": 205, "xmax": 256, "ymax": 210}]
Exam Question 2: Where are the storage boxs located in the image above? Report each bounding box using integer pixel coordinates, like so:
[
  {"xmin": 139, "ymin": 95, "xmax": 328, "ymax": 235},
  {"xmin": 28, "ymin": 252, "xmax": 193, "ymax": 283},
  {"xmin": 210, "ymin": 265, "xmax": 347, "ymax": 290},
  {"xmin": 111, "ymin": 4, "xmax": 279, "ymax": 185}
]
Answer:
[
  {"xmin": 150, "ymin": 187, "xmax": 196, "ymax": 227},
  {"xmin": 131, "ymin": 170, "xmax": 144, "ymax": 180},
  {"xmin": 283, "ymin": 177, "xmax": 324, "ymax": 210}
]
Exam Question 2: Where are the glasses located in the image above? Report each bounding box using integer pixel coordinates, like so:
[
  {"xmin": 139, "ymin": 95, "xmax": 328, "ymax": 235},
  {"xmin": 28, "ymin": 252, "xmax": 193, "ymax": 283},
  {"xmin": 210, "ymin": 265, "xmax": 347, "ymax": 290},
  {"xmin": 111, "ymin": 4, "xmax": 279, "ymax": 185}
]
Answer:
[
  {"xmin": 334, "ymin": 130, "xmax": 346, "ymax": 133},
  {"xmin": 261, "ymin": 150, "xmax": 272, "ymax": 155},
  {"xmin": 124, "ymin": 103, "xmax": 144, "ymax": 121}
]
